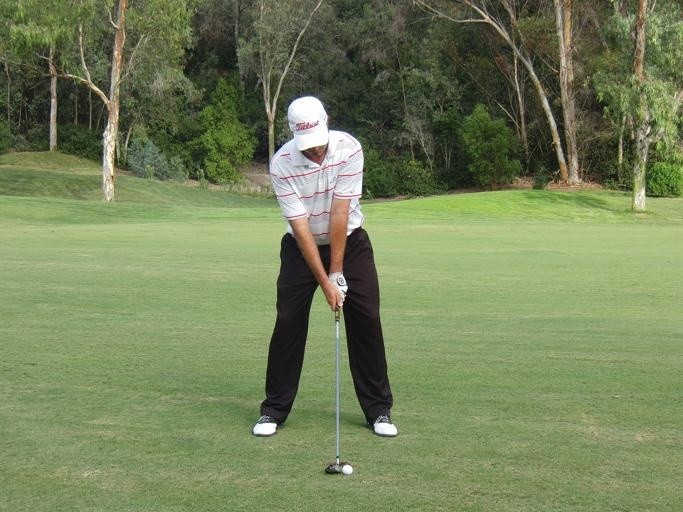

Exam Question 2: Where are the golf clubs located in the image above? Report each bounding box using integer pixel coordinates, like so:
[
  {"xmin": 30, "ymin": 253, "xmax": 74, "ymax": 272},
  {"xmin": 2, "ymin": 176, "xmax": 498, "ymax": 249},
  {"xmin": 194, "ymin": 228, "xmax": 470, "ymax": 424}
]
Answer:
[{"xmin": 325, "ymin": 303, "xmax": 351, "ymax": 472}]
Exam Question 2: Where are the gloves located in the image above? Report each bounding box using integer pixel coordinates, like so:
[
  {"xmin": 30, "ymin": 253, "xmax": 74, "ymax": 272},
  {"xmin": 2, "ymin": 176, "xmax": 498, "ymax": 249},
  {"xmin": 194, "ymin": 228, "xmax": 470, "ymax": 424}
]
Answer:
[{"xmin": 328, "ymin": 273, "xmax": 348, "ymax": 302}]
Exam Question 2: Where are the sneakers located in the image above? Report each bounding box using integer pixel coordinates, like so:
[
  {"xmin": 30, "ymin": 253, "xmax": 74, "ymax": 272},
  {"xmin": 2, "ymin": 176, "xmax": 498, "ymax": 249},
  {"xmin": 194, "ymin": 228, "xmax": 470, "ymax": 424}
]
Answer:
[
  {"xmin": 374, "ymin": 415, "xmax": 397, "ymax": 436},
  {"xmin": 253, "ymin": 415, "xmax": 279, "ymax": 436}
]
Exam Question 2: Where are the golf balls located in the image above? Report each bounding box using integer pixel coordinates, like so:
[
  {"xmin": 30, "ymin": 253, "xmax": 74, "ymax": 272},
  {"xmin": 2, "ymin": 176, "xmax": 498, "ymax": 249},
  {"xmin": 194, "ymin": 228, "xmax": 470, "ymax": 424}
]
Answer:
[{"xmin": 342, "ymin": 465, "xmax": 354, "ymax": 475}]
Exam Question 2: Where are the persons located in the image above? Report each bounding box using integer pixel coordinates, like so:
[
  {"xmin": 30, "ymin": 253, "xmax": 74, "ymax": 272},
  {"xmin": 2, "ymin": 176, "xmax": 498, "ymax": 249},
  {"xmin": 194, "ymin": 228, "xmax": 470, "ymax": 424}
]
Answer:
[{"xmin": 251, "ymin": 96, "xmax": 398, "ymax": 436}]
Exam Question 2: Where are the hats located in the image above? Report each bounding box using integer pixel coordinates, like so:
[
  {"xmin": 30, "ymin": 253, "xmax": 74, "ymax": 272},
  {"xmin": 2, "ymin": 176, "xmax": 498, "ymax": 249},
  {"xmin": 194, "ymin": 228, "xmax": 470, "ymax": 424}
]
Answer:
[{"xmin": 287, "ymin": 96, "xmax": 329, "ymax": 152}]
